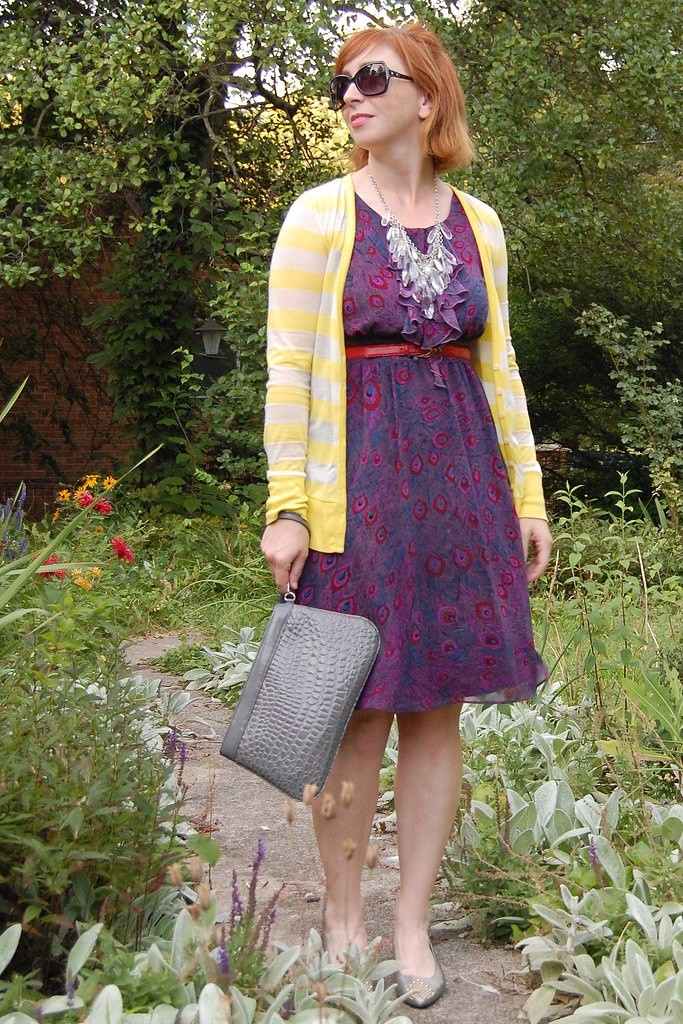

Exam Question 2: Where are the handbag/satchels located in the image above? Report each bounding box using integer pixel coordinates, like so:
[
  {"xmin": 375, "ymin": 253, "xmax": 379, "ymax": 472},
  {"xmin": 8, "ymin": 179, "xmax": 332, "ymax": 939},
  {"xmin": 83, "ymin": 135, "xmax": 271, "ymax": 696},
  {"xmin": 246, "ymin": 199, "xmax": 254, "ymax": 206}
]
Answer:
[{"xmin": 219, "ymin": 512, "xmax": 380, "ymax": 802}]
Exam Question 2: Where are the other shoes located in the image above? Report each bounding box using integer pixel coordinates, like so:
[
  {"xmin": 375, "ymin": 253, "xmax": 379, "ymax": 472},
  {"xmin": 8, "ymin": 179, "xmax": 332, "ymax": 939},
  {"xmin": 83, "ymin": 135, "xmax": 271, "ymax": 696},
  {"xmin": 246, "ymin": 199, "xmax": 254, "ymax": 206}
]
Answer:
[
  {"xmin": 325, "ymin": 967, "xmax": 374, "ymax": 1021},
  {"xmin": 392, "ymin": 936, "xmax": 445, "ymax": 1008}
]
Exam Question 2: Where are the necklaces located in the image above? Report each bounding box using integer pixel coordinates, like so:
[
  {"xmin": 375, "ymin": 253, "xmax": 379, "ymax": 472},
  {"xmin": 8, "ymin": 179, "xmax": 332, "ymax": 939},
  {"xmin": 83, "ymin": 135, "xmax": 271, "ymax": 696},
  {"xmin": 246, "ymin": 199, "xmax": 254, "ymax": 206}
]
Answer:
[{"xmin": 368, "ymin": 172, "xmax": 460, "ymax": 319}]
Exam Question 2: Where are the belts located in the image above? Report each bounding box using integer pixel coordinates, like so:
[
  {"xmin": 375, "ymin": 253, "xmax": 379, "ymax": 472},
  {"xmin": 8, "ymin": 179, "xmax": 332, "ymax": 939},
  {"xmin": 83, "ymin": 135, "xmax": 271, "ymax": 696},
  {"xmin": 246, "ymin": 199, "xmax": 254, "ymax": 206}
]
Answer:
[{"xmin": 345, "ymin": 344, "xmax": 474, "ymax": 361}]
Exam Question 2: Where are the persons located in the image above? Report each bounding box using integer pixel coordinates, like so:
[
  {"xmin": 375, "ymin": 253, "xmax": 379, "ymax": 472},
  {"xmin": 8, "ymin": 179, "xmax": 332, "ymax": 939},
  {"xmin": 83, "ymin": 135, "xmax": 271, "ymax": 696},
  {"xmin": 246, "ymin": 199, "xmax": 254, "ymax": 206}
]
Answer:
[{"xmin": 259, "ymin": 17, "xmax": 553, "ymax": 1010}]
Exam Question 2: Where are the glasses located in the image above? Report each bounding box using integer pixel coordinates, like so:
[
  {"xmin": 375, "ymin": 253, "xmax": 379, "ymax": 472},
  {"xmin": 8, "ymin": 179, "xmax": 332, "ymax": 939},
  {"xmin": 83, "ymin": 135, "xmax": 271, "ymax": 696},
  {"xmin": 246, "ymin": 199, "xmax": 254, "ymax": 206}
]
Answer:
[{"xmin": 327, "ymin": 61, "xmax": 414, "ymax": 111}]
